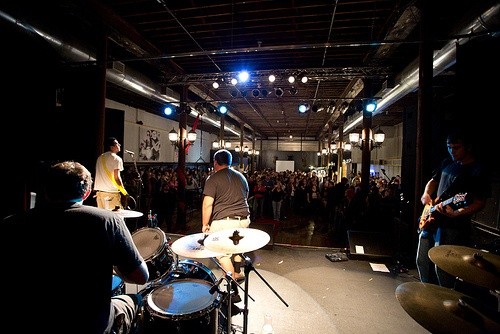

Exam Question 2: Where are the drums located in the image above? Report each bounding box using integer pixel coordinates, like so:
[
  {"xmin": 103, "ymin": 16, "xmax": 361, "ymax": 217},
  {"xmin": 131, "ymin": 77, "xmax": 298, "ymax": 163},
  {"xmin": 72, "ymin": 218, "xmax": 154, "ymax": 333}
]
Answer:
[
  {"xmin": 135, "ymin": 259, "xmax": 221, "ymax": 301},
  {"xmin": 111, "ymin": 273, "xmax": 126, "ymax": 297},
  {"xmin": 130, "ymin": 227, "xmax": 174, "ymax": 282},
  {"xmin": 142, "ymin": 278, "xmax": 220, "ymax": 334}
]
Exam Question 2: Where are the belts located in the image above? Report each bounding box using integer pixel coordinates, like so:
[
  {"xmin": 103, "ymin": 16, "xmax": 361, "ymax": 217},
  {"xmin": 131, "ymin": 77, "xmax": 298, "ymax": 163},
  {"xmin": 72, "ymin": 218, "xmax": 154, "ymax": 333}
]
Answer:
[{"xmin": 224, "ymin": 216, "xmax": 246, "ymax": 220}]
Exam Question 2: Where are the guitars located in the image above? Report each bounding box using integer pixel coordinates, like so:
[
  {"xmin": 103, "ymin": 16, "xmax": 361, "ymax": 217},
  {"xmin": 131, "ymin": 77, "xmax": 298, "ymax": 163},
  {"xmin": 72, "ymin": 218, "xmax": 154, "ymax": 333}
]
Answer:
[
  {"xmin": 121, "ymin": 193, "xmax": 136, "ymax": 210},
  {"xmin": 419, "ymin": 191, "xmax": 468, "ymax": 231}
]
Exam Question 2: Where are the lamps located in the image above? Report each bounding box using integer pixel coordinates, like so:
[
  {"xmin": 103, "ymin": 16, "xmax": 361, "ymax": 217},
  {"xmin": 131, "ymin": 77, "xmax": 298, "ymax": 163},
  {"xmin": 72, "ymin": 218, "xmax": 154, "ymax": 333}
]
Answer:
[
  {"xmin": 312, "ymin": 103, "xmax": 325, "ymax": 113},
  {"xmin": 350, "ymin": 99, "xmax": 365, "ymax": 114},
  {"xmin": 235, "ymin": 145, "xmax": 261, "ymax": 158},
  {"xmin": 364, "ymin": 98, "xmax": 376, "ymax": 113},
  {"xmin": 185, "ymin": 128, "xmax": 197, "ymax": 149},
  {"xmin": 212, "ymin": 138, "xmax": 232, "ymax": 151},
  {"xmin": 317, "ymin": 124, "xmax": 385, "ymax": 159},
  {"xmin": 169, "ymin": 127, "xmax": 178, "ymax": 146},
  {"xmin": 338, "ymin": 101, "xmax": 349, "ymax": 113},
  {"xmin": 211, "ymin": 68, "xmax": 311, "ymax": 100},
  {"xmin": 163, "ymin": 101, "xmax": 230, "ymax": 118},
  {"xmin": 326, "ymin": 100, "xmax": 338, "ymax": 114},
  {"xmin": 298, "ymin": 101, "xmax": 310, "ymax": 113}
]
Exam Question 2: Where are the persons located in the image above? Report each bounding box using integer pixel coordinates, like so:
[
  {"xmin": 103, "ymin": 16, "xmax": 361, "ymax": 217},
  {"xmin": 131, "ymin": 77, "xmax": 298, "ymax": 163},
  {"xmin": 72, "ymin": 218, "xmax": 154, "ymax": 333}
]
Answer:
[
  {"xmin": 201, "ymin": 149, "xmax": 251, "ymax": 283},
  {"xmin": 94, "ymin": 137, "xmax": 127, "ymax": 209},
  {"xmin": 0, "ymin": 160, "xmax": 149, "ymax": 334},
  {"xmin": 123, "ymin": 168, "xmax": 404, "ymax": 245},
  {"xmin": 415, "ymin": 129, "xmax": 491, "ymax": 289}
]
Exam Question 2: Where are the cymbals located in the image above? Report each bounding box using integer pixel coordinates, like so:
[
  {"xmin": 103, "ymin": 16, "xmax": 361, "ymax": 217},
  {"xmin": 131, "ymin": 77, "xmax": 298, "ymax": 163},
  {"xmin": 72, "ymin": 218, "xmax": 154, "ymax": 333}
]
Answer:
[
  {"xmin": 428, "ymin": 244, "xmax": 500, "ymax": 288},
  {"xmin": 204, "ymin": 228, "xmax": 271, "ymax": 255},
  {"xmin": 394, "ymin": 281, "xmax": 500, "ymax": 334},
  {"xmin": 105, "ymin": 208, "xmax": 143, "ymax": 218},
  {"xmin": 171, "ymin": 231, "xmax": 218, "ymax": 258}
]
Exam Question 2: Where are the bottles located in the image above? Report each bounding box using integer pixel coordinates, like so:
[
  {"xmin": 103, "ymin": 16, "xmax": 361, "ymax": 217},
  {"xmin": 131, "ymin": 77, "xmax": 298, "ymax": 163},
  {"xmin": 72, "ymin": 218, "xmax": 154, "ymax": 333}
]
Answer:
[{"xmin": 147, "ymin": 210, "xmax": 157, "ymax": 228}]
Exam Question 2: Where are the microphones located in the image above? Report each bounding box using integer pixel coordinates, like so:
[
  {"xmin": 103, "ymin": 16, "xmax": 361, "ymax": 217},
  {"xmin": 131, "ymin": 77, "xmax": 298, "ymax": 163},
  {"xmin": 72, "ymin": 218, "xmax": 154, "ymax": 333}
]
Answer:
[
  {"xmin": 209, "ymin": 278, "xmax": 224, "ymax": 295},
  {"xmin": 125, "ymin": 150, "xmax": 134, "ymax": 155}
]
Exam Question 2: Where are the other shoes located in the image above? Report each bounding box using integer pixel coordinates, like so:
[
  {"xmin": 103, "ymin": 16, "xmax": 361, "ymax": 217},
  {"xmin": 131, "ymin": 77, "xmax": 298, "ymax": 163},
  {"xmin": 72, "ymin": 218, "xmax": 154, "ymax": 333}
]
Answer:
[
  {"xmin": 236, "ymin": 274, "xmax": 244, "ymax": 283},
  {"xmin": 231, "ymin": 291, "xmax": 242, "ymax": 303}
]
